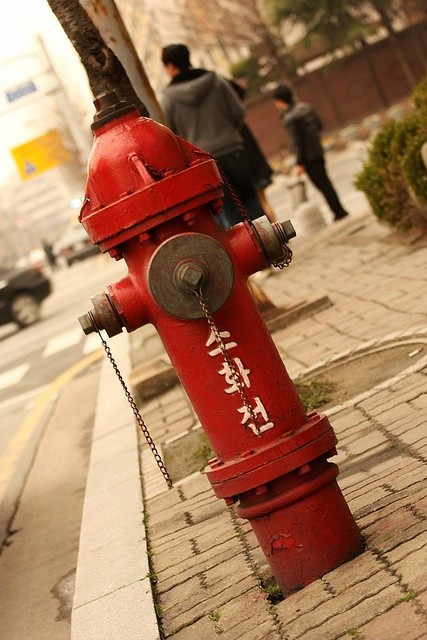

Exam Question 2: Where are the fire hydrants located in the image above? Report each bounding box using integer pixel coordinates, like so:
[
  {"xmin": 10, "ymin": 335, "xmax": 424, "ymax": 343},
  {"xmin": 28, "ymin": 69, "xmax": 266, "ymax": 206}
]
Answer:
[{"xmin": 72, "ymin": 89, "xmax": 367, "ymax": 601}]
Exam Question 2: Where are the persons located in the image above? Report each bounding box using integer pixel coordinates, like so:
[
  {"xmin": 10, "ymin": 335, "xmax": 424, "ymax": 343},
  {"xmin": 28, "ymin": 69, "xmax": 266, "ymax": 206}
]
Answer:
[
  {"xmin": 223, "ymin": 77, "xmax": 277, "ymax": 225},
  {"xmin": 272, "ymin": 84, "xmax": 349, "ymax": 221},
  {"xmin": 160, "ymin": 42, "xmax": 264, "ymax": 225}
]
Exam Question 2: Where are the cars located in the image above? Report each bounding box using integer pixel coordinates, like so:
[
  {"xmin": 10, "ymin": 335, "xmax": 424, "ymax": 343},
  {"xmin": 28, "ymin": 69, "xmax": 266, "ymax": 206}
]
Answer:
[
  {"xmin": 1, "ymin": 269, "xmax": 50, "ymax": 332},
  {"xmin": 61, "ymin": 227, "xmax": 100, "ymax": 267}
]
